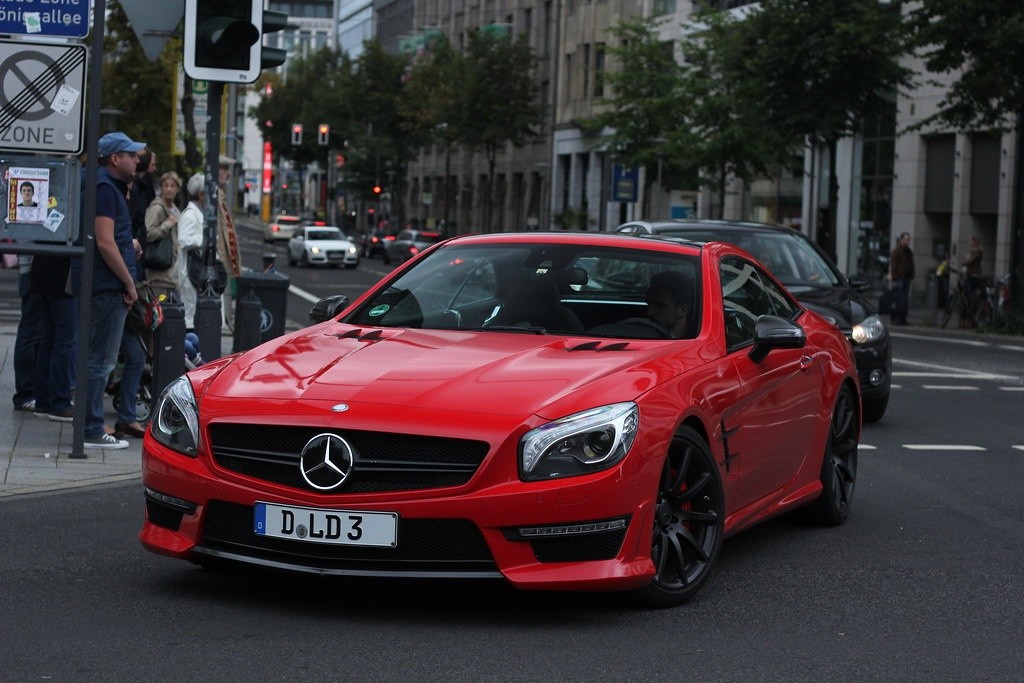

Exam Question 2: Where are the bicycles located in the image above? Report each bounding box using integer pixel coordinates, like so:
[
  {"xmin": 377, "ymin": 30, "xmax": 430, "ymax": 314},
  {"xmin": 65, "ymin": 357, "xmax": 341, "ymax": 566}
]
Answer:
[{"xmin": 939, "ymin": 262, "xmax": 994, "ymax": 329}]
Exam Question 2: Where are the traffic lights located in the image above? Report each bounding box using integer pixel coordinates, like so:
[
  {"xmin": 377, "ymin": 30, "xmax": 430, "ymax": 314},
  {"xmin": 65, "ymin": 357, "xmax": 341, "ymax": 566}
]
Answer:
[
  {"xmin": 374, "ymin": 186, "xmax": 381, "ymax": 193},
  {"xmin": 319, "ymin": 124, "xmax": 329, "ymax": 144},
  {"xmin": 184, "ymin": 0, "xmax": 289, "ymax": 83},
  {"xmin": 292, "ymin": 123, "xmax": 303, "ymax": 144}
]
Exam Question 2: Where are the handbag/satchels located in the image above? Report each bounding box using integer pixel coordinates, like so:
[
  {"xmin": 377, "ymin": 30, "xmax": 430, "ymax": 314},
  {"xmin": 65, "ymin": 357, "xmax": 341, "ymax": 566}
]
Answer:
[
  {"xmin": 140, "ymin": 202, "xmax": 174, "ymax": 269},
  {"xmin": 187, "ymin": 248, "xmax": 228, "ymax": 297}
]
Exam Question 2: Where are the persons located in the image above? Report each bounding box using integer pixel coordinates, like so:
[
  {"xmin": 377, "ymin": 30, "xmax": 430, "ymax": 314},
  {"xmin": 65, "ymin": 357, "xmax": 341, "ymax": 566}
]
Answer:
[
  {"xmin": 643, "ymin": 271, "xmax": 693, "ymax": 340},
  {"xmin": 14, "ymin": 132, "xmax": 240, "ymax": 449},
  {"xmin": 888, "ymin": 231, "xmax": 914, "ymax": 325},
  {"xmin": 16, "ymin": 182, "xmax": 39, "ymax": 207},
  {"xmin": 963, "ymin": 235, "xmax": 986, "ymax": 279}
]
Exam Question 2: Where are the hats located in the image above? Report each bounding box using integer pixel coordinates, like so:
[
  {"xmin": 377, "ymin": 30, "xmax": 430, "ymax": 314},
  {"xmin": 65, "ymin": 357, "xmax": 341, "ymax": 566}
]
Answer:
[
  {"xmin": 219, "ymin": 155, "xmax": 235, "ymax": 165},
  {"xmin": 98, "ymin": 131, "xmax": 146, "ymax": 157}
]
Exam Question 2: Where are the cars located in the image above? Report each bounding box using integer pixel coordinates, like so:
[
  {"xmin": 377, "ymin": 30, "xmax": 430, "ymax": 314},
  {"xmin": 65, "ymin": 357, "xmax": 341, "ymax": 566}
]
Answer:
[
  {"xmin": 288, "ymin": 228, "xmax": 361, "ymax": 269},
  {"xmin": 588, "ymin": 219, "xmax": 893, "ymax": 423},
  {"xmin": 137, "ymin": 232, "xmax": 864, "ymax": 609},
  {"xmin": 264, "ymin": 216, "xmax": 301, "ymax": 243},
  {"xmin": 383, "ymin": 230, "xmax": 442, "ymax": 267},
  {"xmin": 360, "ymin": 228, "xmax": 398, "ymax": 258}
]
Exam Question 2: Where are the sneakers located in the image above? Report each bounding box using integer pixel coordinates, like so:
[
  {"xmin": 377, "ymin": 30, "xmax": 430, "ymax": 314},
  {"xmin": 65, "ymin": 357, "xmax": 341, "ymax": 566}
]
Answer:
[{"xmin": 84, "ymin": 431, "xmax": 129, "ymax": 449}]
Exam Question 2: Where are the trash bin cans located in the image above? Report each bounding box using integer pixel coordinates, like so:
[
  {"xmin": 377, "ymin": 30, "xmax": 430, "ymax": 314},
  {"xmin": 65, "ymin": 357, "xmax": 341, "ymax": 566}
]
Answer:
[
  {"xmin": 927, "ymin": 270, "xmax": 950, "ymax": 306},
  {"xmin": 233, "ymin": 272, "xmax": 290, "ymax": 352}
]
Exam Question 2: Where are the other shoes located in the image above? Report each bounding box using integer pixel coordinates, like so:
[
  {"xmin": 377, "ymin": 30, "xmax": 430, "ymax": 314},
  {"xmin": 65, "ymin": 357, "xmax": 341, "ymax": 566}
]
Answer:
[
  {"xmin": 108, "ymin": 421, "xmax": 145, "ymax": 439},
  {"xmin": 22, "ymin": 399, "xmax": 73, "ymax": 421}
]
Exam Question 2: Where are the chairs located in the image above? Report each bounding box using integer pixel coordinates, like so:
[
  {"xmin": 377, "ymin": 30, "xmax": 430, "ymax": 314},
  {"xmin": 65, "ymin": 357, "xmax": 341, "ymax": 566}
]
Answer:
[
  {"xmin": 621, "ymin": 271, "xmax": 686, "ymax": 337},
  {"xmin": 487, "ymin": 274, "xmax": 583, "ymax": 336}
]
